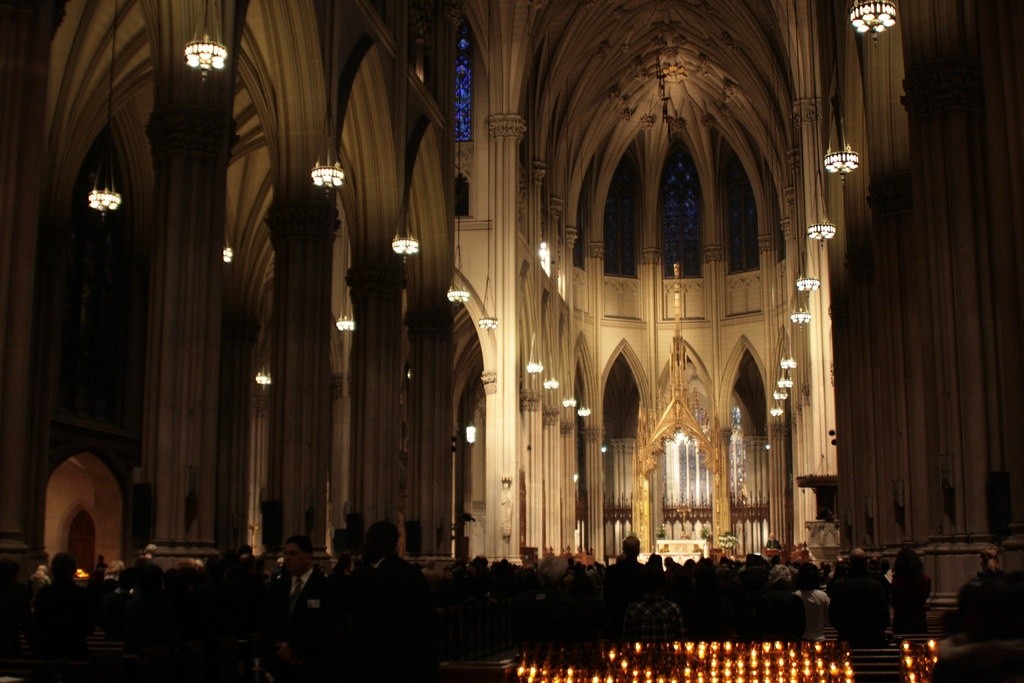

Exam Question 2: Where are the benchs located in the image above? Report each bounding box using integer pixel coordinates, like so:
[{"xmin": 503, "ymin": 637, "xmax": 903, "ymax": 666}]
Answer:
[{"xmin": 0, "ymin": 588, "xmax": 966, "ymax": 683}]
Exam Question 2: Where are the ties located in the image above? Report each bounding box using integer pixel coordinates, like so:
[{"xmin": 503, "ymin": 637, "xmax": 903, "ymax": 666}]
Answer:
[{"xmin": 289, "ymin": 577, "xmax": 304, "ymax": 617}]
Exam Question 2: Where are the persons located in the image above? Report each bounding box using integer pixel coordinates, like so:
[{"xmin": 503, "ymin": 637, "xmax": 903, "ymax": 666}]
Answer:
[
  {"xmin": 0, "ymin": 520, "xmax": 1024, "ymax": 683},
  {"xmin": 891, "ymin": 548, "xmax": 931, "ymax": 634},
  {"xmin": 622, "ymin": 573, "xmax": 689, "ymax": 642}
]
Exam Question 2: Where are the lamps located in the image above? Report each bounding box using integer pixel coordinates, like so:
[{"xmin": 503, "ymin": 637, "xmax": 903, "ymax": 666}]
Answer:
[
  {"xmin": 577, "ymin": 380, "xmax": 592, "ymax": 417},
  {"xmin": 768, "ymin": 0, "xmax": 899, "ymax": 420},
  {"xmin": 477, "ymin": 0, "xmax": 500, "ymax": 336},
  {"xmin": 525, "ymin": 328, "xmax": 544, "ymax": 377},
  {"xmin": 309, "ymin": 0, "xmax": 346, "ymax": 201},
  {"xmin": 542, "ymin": 363, "xmax": 559, "ymax": 391},
  {"xmin": 391, "ymin": 183, "xmax": 420, "ymax": 265},
  {"xmin": 562, "ymin": 368, "xmax": 577, "ymax": 409},
  {"xmin": 183, "ymin": 0, "xmax": 228, "ymax": 86},
  {"xmin": 87, "ymin": 0, "xmax": 124, "ymax": 221},
  {"xmin": 255, "ymin": 351, "xmax": 272, "ymax": 391},
  {"xmin": 335, "ymin": 221, "xmax": 356, "ymax": 333},
  {"xmin": 445, "ymin": 65, "xmax": 472, "ymax": 308}
]
